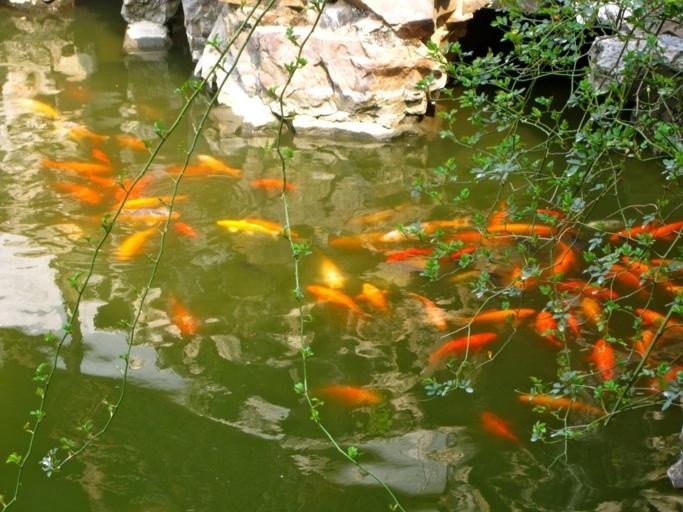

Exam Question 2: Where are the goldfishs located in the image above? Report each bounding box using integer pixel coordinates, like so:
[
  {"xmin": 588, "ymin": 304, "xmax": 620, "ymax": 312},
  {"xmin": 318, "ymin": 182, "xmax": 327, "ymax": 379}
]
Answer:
[
  {"xmin": 0, "ymin": 87, "xmax": 245, "ymax": 262},
  {"xmin": 304, "ymin": 186, "xmax": 683, "ymax": 393}
]
